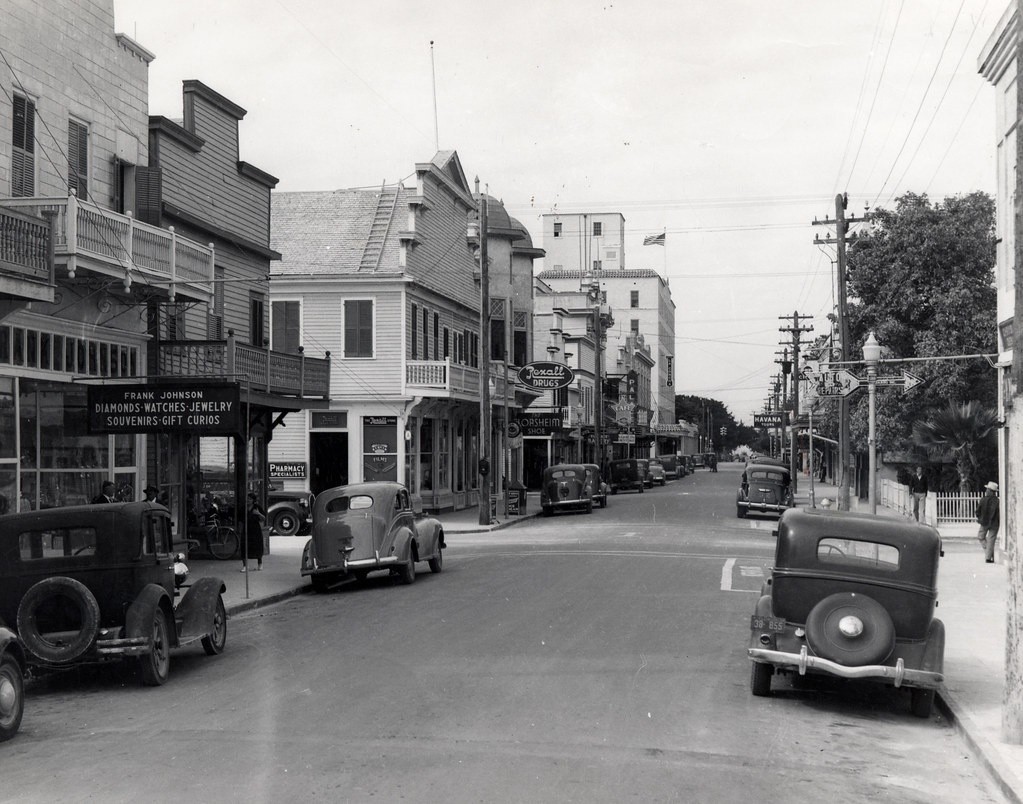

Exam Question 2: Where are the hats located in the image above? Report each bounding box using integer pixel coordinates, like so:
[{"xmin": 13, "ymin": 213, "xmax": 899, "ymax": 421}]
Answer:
[
  {"xmin": 984, "ymin": 481, "xmax": 999, "ymax": 491},
  {"xmin": 143, "ymin": 484, "xmax": 159, "ymax": 493}
]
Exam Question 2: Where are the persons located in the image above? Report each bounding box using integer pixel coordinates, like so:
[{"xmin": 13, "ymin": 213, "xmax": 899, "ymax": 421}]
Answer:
[
  {"xmin": 708, "ymin": 454, "xmax": 714, "ymax": 472},
  {"xmin": 97, "ymin": 481, "xmax": 119, "ymax": 503},
  {"xmin": 975, "ymin": 481, "xmax": 1001, "ymax": 563},
  {"xmin": 908, "ymin": 465, "xmax": 928, "ymax": 523},
  {"xmin": 237, "ymin": 493, "xmax": 267, "ymax": 573},
  {"xmin": 143, "ymin": 485, "xmax": 166, "ymax": 506},
  {"xmin": 711, "ymin": 455, "xmax": 717, "ymax": 472}
]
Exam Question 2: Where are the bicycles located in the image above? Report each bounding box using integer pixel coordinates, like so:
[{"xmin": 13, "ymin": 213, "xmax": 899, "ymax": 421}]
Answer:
[{"xmin": 188, "ymin": 511, "xmax": 239, "ymax": 560}]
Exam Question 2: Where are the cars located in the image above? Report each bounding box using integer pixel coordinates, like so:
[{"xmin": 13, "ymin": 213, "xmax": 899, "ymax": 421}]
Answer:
[
  {"xmin": 196, "ymin": 465, "xmax": 318, "ymax": 537},
  {"xmin": 745, "ymin": 506, "xmax": 946, "ymax": 718},
  {"xmin": 582, "ymin": 463, "xmax": 607, "ymax": 509},
  {"xmin": 677, "ymin": 455, "xmax": 694, "ymax": 475},
  {"xmin": 541, "ymin": 464, "xmax": 592, "ymax": 518},
  {"xmin": 637, "ymin": 459, "xmax": 654, "ymax": 488},
  {"xmin": 691, "ymin": 454, "xmax": 706, "ymax": 468},
  {"xmin": 648, "ymin": 458, "xmax": 667, "ymax": 486},
  {"xmin": 658, "ymin": 454, "xmax": 682, "ymax": 480},
  {"xmin": 300, "ymin": 481, "xmax": 447, "ymax": 593},
  {"xmin": 0, "ymin": 501, "xmax": 228, "ymax": 686},
  {"xmin": 608, "ymin": 459, "xmax": 644, "ymax": 494},
  {"xmin": 735, "ymin": 464, "xmax": 794, "ymax": 517}
]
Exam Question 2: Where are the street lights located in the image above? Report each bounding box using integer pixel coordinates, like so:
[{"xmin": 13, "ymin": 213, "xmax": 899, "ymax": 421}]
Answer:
[
  {"xmin": 576, "ymin": 402, "xmax": 583, "ymax": 464},
  {"xmin": 710, "ymin": 439, "xmax": 712, "ymax": 454},
  {"xmin": 627, "ymin": 417, "xmax": 631, "ymax": 458},
  {"xmin": 699, "ymin": 435, "xmax": 702, "ymax": 454},
  {"xmin": 862, "ymin": 332, "xmax": 883, "ymax": 514},
  {"xmin": 705, "ymin": 437, "xmax": 707, "ymax": 453},
  {"xmin": 803, "ymin": 392, "xmax": 818, "ymax": 509}
]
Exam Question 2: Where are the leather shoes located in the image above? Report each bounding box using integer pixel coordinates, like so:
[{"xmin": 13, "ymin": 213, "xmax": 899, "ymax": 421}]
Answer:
[{"xmin": 986, "ymin": 558, "xmax": 994, "ymax": 562}]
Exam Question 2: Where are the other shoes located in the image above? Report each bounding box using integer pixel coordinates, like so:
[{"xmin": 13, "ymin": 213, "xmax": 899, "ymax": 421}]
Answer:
[
  {"xmin": 256, "ymin": 564, "xmax": 263, "ymax": 570},
  {"xmin": 240, "ymin": 566, "xmax": 248, "ymax": 572}
]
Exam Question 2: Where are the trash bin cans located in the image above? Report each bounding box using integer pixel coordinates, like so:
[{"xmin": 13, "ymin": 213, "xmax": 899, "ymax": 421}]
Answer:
[{"xmin": 507, "ymin": 480, "xmax": 527, "ymax": 514}]
